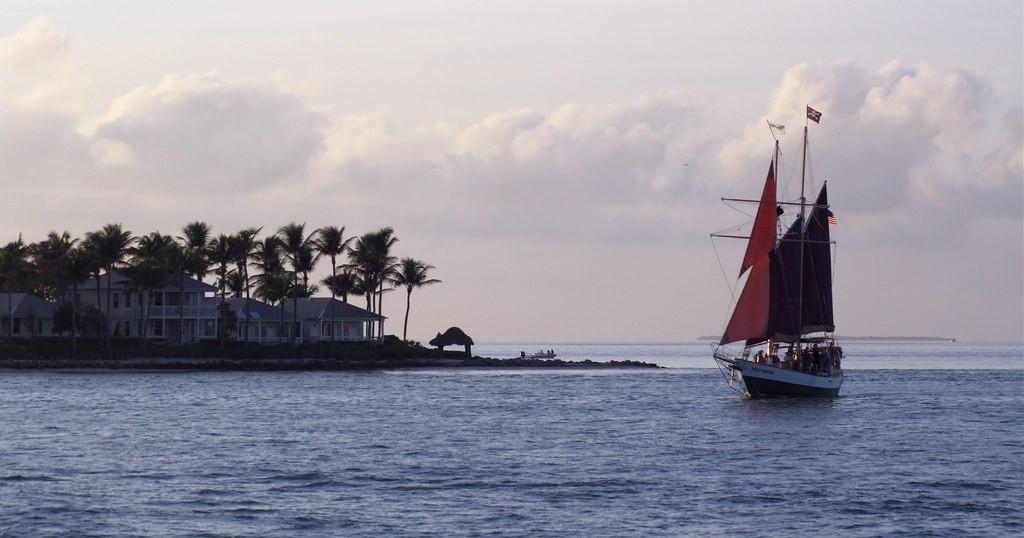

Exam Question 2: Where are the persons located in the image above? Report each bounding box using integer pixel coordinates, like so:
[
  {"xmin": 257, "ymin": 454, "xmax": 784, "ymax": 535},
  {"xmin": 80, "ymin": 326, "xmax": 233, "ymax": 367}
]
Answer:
[
  {"xmin": 541, "ymin": 349, "xmax": 554, "ymax": 355},
  {"xmin": 754, "ymin": 343, "xmax": 842, "ymax": 373}
]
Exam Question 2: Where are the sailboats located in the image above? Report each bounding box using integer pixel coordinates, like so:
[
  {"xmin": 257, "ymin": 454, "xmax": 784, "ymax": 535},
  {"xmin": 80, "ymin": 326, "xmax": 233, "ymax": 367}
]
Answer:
[{"xmin": 708, "ymin": 103, "xmax": 847, "ymax": 400}]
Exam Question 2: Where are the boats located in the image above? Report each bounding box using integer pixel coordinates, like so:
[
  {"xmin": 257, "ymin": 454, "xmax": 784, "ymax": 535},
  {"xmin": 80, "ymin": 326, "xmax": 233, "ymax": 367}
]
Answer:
[{"xmin": 525, "ymin": 353, "xmax": 557, "ymax": 359}]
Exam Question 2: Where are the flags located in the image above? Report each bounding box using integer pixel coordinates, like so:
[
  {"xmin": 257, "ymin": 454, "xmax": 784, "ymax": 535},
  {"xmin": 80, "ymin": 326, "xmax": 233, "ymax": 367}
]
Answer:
[
  {"xmin": 828, "ymin": 215, "xmax": 836, "ymax": 224},
  {"xmin": 767, "ymin": 120, "xmax": 786, "ymax": 135},
  {"xmin": 807, "ymin": 105, "xmax": 822, "ymax": 124}
]
